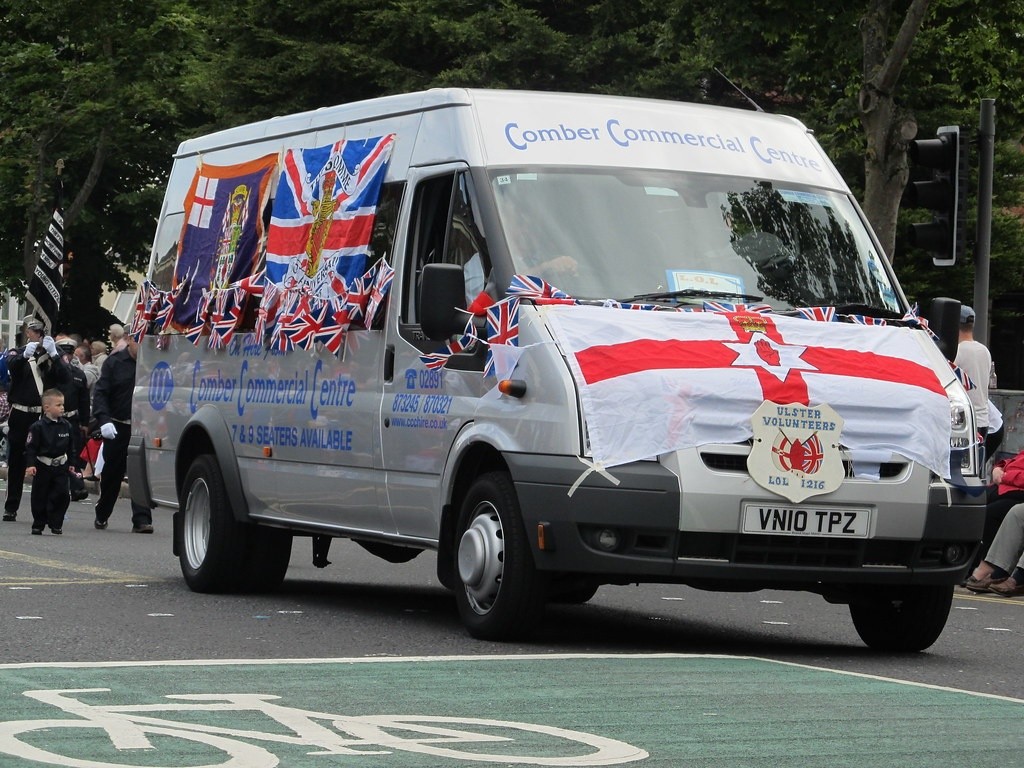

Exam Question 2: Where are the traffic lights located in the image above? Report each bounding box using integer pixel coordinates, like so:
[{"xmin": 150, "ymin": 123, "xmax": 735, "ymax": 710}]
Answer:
[{"xmin": 906, "ymin": 125, "xmax": 959, "ymax": 267}]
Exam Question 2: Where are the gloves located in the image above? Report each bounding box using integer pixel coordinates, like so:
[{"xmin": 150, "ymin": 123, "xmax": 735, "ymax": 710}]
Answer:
[
  {"xmin": 100, "ymin": 423, "xmax": 118, "ymax": 439},
  {"xmin": 23, "ymin": 341, "xmax": 39, "ymax": 359},
  {"xmin": 42, "ymin": 336, "xmax": 57, "ymax": 357}
]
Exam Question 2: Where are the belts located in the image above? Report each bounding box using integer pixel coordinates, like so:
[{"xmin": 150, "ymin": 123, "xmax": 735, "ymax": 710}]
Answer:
[
  {"xmin": 12, "ymin": 403, "xmax": 42, "ymax": 413},
  {"xmin": 37, "ymin": 452, "xmax": 68, "ymax": 466},
  {"xmin": 44, "ymin": 410, "xmax": 78, "ymax": 417},
  {"xmin": 110, "ymin": 417, "xmax": 131, "ymax": 424}
]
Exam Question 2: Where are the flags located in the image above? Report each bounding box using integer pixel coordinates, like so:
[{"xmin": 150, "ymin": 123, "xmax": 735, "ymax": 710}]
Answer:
[
  {"xmin": 129, "ymin": 133, "xmax": 396, "ymax": 362},
  {"xmin": 421, "ymin": 276, "xmax": 976, "ymax": 479},
  {"xmin": 22, "ymin": 203, "xmax": 64, "ymax": 336}
]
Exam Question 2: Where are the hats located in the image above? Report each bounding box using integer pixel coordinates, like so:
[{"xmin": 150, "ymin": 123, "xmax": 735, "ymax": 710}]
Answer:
[
  {"xmin": 26, "ymin": 320, "xmax": 44, "ymax": 330},
  {"xmin": 55, "ymin": 339, "xmax": 77, "ymax": 353},
  {"xmin": 123, "ymin": 323, "xmax": 131, "ymax": 334},
  {"xmin": 959, "ymin": 305, "xmax": 976, "ymax": 323}
]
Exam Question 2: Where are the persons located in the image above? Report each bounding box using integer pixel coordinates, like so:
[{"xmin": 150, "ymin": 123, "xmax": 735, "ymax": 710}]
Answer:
[
  {"xmin": 978, "ymin": 398, "xmax": 1004, "ymax": 478},
  {"xmin": 464, "ymin": 199, "xmax": 579, "ymax": 312},
  {"xmin": 966, "ymin": 503, "xmax": 1024, "ymax": 598},
  {"xmin": 19, "ymin": 388, "xmax": 77, "ymax": 535},
  {"xmin": 93, "ymin": 333, "xmax": 158, "ymax": 534},
  {"xmin": 953, "ymin": 305, "xmax": 991, "ymax": 447},
  {"xmin": 0, "ymin": 314, "xmax": 129, "ymax": 522},
  {"xmin": 960, "ymin": 450, "xmax": 1024, "ymax": 588}
]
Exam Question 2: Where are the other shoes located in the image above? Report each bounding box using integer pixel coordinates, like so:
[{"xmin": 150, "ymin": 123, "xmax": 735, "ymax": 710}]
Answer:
[
  {"xmin": 989, "ymin": 578, "xmax": 1024, "ymax": 597},
  {"xmin": 133, "ymin": 522, "xmax": 154, "ymax": 533},
  {"xmin": 32, "ymin": 527, "xmax": 42, "ymax": 535},
  {"xmin": 967, "ymin": 575, "xmax": 1008, "ymax": 591},
  {"xmin": 3, "ymin": 509, "xmax": 17, "ymax": 520},
  {"xmin": 71, "ymin": 490, "xmax": 88, "ymax": 501},
  {"xmin": 94, "ymin": 504, "xmax": 108, "ymax": 528},
  {"xmin": 82, "ymin": 470, "xmax": 93, "ymax": 478},
  {"xmin": 48, "ymin": 524, "xmax": 62, "ymax": 534},
  {"xmin": 960, "ymin": 576, "xmax": 978, "ymax": 587},
  {"xmin": 88, "ymin": 475, "xmax": 100, "ymax": 481}
]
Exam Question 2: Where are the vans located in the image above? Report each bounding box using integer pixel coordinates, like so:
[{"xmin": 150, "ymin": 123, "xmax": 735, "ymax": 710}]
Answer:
[{"xmin": 126, "ymin": 86, "xmax": 985, "ymax": 656}]
